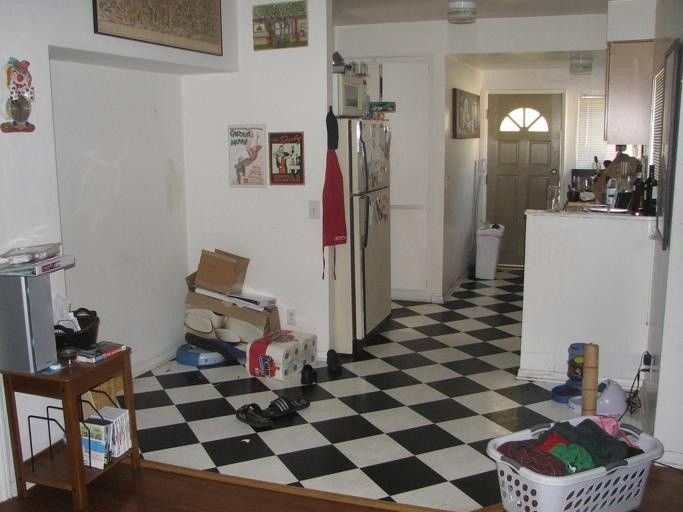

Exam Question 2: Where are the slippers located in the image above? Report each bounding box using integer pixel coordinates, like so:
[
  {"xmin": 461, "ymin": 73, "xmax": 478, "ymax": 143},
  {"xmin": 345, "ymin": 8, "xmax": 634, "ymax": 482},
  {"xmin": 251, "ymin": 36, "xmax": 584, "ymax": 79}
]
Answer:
[
  {"xmin": 236, "ymin": 403, "xmax": 273, "ymax": 428},
  {"xmin": 264, "ymin": 395, "xmax": 310, "ymax": 418}
]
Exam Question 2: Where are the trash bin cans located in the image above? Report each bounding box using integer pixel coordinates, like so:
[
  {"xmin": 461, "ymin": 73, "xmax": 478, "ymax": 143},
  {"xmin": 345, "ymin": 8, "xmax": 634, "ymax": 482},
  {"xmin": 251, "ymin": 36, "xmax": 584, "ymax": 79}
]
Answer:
[{"xmin": 475, "ymin": 224, "xmax": 505, "ymax": 280}]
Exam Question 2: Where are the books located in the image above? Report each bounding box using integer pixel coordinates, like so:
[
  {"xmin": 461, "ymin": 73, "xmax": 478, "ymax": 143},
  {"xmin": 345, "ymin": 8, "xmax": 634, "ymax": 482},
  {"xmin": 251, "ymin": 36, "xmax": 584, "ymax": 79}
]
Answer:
[
  {"xmin": 0, "ymin": 241, "xmax": 76, "ymax": 280},
  {"xmin": 74, "ymin": 340, "xmax": 126, "ymax": 364},
  {"xmin": 76, "ymin": 405, "xmax": 132, "ymax": 469}
]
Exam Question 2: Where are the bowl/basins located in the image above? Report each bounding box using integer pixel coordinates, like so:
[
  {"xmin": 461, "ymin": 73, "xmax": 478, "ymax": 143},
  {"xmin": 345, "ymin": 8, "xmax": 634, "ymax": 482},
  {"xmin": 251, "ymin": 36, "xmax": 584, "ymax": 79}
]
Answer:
[{"xmin": 551, "ymin": 385, "xmax": 582, "ymax": 403}]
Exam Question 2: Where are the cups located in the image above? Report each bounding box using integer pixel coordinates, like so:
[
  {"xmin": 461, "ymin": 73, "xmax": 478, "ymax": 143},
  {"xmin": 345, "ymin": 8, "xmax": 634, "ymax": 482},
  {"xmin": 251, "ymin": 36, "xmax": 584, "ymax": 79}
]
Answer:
[
  {"xmin": 567, "ymin": 191, "xmax": 577, "ymax": 203},
  {"xmin": 56, "ymin": 346, "xmax": 79, "ymax": 368},
  {"xmin": 592, "ymin": 163, "xmax": 601, "ymax": 174},
  {"xmin": 616, "ymin": 192, "xmax": 633, "ymax": 211}
]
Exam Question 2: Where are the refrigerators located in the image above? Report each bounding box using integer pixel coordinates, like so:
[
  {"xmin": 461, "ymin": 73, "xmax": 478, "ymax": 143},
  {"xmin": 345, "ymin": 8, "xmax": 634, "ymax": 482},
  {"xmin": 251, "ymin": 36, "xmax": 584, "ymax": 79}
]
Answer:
[{"xmin": 332, "ymin": 116, "xmax": 393, "ymax": 348}]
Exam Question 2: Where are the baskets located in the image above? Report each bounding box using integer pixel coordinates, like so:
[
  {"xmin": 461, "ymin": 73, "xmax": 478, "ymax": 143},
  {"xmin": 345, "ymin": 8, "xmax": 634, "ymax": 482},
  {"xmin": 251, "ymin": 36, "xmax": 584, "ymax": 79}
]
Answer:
[{"xmin": 485, "ymin": 415, "xmax": 664, "ymax": 512}]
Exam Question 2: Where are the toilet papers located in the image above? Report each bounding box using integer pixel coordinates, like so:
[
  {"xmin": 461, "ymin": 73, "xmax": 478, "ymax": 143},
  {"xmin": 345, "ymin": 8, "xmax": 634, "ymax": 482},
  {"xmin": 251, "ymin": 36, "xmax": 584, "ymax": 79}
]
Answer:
[{"xmin": 245, "ymin": 329, "xmax": 318, "ymax": 381}]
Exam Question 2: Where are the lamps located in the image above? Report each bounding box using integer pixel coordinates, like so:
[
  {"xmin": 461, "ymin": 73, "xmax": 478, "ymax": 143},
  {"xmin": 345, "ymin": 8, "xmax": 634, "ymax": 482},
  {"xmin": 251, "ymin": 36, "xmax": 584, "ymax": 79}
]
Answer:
[
  {"xmin": 446, "ymin": 2, "xmax": 478, "ymax": 26},
  {"xmin": 569, "ymin": 57, "xmax": 593, "ymax": 75}
]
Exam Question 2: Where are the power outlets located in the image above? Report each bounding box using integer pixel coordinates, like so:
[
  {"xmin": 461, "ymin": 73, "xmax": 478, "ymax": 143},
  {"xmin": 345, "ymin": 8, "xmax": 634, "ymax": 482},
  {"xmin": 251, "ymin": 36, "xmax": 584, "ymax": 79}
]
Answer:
[
  {"xmin": 643, "ymin": 359, "xmax": 652, "ymax": 380},
  {"xmin": 287, "ymin": 309, "xmax": 297, "ymax": 326}
]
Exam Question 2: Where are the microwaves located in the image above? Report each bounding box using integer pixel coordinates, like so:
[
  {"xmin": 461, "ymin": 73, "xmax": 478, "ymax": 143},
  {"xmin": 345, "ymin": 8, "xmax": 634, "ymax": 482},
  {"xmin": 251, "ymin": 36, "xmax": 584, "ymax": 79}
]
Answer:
[{"xmin": 331, "ymin": 73, "xmax": 367, "ymax": 118}]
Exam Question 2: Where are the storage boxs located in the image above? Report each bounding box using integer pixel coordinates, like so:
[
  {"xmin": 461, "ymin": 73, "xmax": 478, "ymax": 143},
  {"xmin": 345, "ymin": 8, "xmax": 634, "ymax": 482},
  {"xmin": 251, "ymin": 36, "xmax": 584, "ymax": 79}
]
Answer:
[
  {"xmin": 184, "ymin": 270, "xmax": 282, "ymax": 334},
  {"xmin": 194, "ymin": 246, "xmax": 251, "ymax": 295}
]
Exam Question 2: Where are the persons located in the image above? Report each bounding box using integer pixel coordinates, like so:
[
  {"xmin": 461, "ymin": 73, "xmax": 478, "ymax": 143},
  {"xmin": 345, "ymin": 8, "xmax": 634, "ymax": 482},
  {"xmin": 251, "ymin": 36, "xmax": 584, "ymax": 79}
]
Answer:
[{"xmin": 272, "ymin": 146, "xmax": 293, "ymax": 175}]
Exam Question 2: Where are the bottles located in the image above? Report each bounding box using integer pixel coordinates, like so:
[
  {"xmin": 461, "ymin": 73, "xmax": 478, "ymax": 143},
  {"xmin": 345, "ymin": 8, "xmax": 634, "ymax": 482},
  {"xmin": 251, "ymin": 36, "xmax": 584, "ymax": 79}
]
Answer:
[
  {"xmin": 632, "ymin": 164, "xmax": 658, "ymax": 217},
  {"xmin": 575, "ymin": 174, "xmax": 598, "ymax": 192},
  {"xmin": 545, "ymin": 184, "xmax": 560, "ymax": 212}
]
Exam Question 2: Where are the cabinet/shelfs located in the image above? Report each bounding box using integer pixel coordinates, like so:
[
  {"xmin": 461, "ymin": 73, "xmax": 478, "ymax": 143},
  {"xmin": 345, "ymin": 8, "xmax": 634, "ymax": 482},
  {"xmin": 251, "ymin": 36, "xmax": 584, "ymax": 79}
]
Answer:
[
  {"xmin": 601, "ymin": 40, "xmax": 653, "ymax": 145},
  {"xmin": 0, "ymin": 341, "xmax": 144, "ymax": 511}
]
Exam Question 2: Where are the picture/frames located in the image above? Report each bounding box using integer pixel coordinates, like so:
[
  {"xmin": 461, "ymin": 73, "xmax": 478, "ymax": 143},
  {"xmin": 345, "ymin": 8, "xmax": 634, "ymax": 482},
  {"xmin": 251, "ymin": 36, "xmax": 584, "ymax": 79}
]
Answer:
[{"xmin": 654, "ymin": 38, "xmax": 681, "ymax": 250}]
